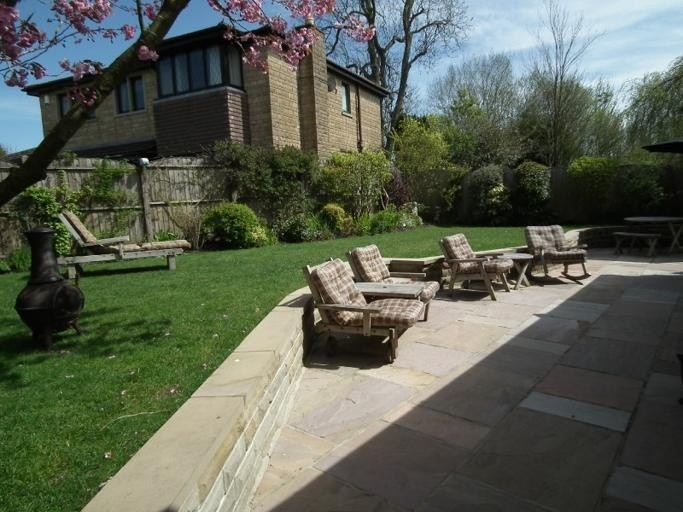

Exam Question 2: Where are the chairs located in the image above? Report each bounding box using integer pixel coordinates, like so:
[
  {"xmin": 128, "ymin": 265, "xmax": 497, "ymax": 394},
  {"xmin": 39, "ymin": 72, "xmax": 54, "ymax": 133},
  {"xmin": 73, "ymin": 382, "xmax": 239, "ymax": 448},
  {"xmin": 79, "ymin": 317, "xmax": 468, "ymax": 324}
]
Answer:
[
  {"xmin": 301, "ymin": 244, "xmax": 441, "ymax": 365},
  {"xmin": 526, "ymin": 223, "xmax": 592, "ymax": 283},
  {"xmin": 439, "ymin": 231, "xmax": 514, "ymax": 300},
  {"xmin": 55, "ymin": 209, "xmax": 191, "ymax": 280}
]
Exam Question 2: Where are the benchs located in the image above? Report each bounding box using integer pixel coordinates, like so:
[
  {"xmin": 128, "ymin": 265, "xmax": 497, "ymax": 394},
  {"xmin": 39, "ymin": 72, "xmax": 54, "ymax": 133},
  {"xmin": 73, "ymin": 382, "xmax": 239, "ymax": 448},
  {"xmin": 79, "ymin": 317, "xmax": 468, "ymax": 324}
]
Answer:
[{"xmin": 611, "ymin": 231, "xmax": 662, "ymax": 257}]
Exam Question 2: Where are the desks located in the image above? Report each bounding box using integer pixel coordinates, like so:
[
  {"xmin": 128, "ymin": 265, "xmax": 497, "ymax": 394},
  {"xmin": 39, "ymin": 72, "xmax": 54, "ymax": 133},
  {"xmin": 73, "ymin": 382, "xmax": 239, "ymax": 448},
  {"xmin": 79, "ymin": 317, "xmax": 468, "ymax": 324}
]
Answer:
[{"xmin": 623, "ymin": 216, "xmax": 683, "ymax": 256}]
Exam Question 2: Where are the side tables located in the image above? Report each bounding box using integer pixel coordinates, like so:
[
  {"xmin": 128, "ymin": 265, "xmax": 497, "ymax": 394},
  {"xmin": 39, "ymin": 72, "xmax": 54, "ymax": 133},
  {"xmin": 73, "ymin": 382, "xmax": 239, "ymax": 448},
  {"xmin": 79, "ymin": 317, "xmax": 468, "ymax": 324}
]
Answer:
[{"xmin": 495, "ymin": 251, "xmax": 534, "ymax": 290}]
